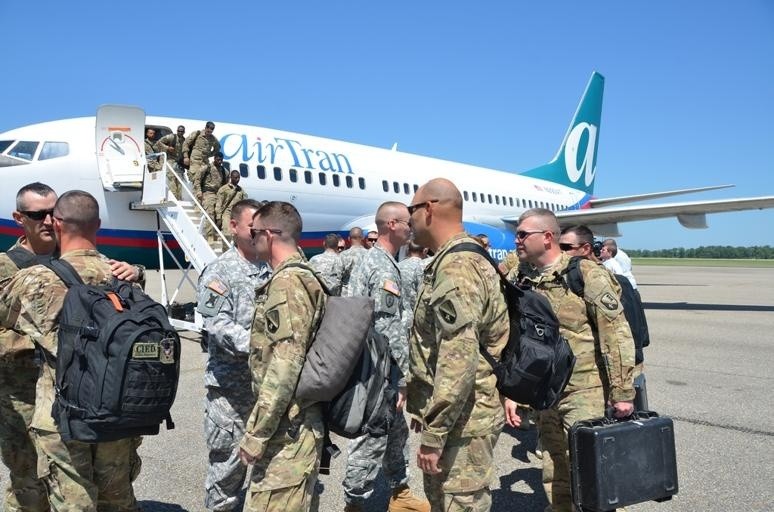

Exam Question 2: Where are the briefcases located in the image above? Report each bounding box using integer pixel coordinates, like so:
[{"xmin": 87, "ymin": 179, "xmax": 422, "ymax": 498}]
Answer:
[
  {"xmin": 569, "ymin": 407, "xmax": 678, "ymax": 511},
  {"xmin": 633, "ymin": 373, "xmax": 648, "ymax": 411}
]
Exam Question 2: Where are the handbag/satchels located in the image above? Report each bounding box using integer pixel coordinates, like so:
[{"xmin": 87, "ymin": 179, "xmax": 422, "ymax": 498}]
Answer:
[{"xmin": 294, "ymin": 295, "xmax": 374, "ymax": 403}]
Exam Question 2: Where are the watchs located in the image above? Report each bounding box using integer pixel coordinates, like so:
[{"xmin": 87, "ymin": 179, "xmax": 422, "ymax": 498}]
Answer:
[{"xmin": 132, "ymin": 262, "xmax": 144, "ymax": 283}]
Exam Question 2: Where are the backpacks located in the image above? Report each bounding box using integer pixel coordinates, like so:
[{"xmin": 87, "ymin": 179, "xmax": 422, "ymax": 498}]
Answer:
[
  {"xmin": 569, "ymin": 255, "xmax": 649, "ymax": 368},
  {"xmin": 323, "ymin": 332, "xmax": 403, "ymax": 438},
  {"xmin": 44, "ymin": 257, "xmax": 181, "ymax": 444},
  {"xmin": 433, "ymin": 242, "xmax": 576, "ymax": 411}
]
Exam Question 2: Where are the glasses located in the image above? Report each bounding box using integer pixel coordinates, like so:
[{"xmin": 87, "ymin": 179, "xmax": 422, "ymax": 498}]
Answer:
[
  {"xmin": 250, "ymin": 228, "xmax": 281, "ymax": 238},
  {"xmin": 368, "ymin": 238, "xmax": 377, "ymax": 242},
  {"xmin": 517, "ymin": 231, "xmax": 545, "ymax": 238},
  {"xmin": 559, "ymin": 244, "xmax": 584, "ymax": 250},
  {"xmin": 337, "ymin": 247, "xmax": 344, "ymax": 249},
  {"xmin": 20, "ymin": 211, "xmax": 54, "ymax": 220},
  {"xmin": 407, "ymin": 200, "xmax": 439, "ymax": 215}
]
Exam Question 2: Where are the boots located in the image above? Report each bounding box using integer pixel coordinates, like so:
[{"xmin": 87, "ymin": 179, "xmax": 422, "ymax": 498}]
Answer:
[
  {"xmin": 388, "ymin": 483, "xmax": 431, "ymax": 512},
  {"xmin": 517, "ymin": 409, "xmax": 530, "ymax": 430}
]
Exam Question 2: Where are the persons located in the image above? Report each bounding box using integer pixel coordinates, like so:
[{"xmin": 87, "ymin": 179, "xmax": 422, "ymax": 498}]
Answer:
[
  {"xmin": 598, "ymin": 238, "xmax": 638, "ymax": 290},
  {"xmin": 309, "ymin": 227, "xmax": 379, "ymax": 297},
  {"xmin": 342, "ymin": 201, "xmax": 435, "ymax": 512},
  {"xmin": 403, "ymin": 177, "xmax": 513, "ymax": 511},
  {"xmin": 197, "ymin": 198, "xmax": 273, "ymax": 512},
  {"xmin": 397, "ymin": 240, "xmax": 429, "ymax": 299},
  {"xmin": 236, "ymin": 200, "xmax": 329, "ymax": 512},
  {"xmin": 477, "ymin": 234, "xmax": 533, "ymax": 284},
  {"xmin": 144, "ymin": 121, "xmax": 248, "ymax": 255},
  {"xmin": 557, "ymin": 218, "xmax": 653, "ymax": 415},
  {"xmin": 499, "ymin": 206, "xmax": 639, "ymax": 512}
]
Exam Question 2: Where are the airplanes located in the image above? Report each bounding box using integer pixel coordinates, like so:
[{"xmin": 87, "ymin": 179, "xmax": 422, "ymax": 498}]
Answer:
[{"xmin": 0, "ymin": 71, "xmax": 774, "ymax": 338}]
